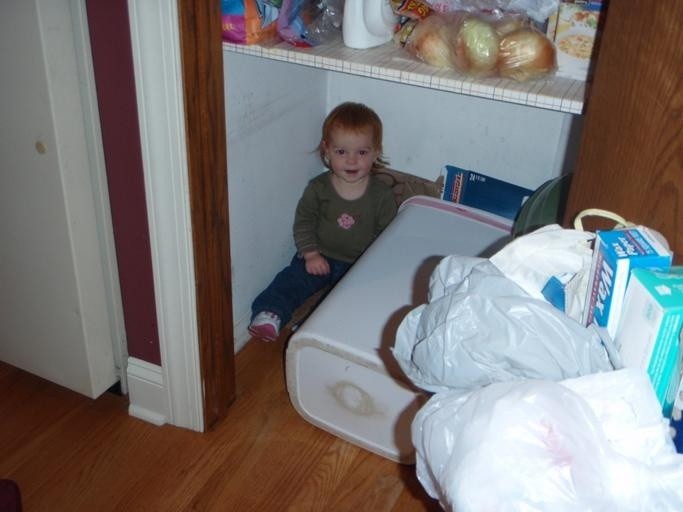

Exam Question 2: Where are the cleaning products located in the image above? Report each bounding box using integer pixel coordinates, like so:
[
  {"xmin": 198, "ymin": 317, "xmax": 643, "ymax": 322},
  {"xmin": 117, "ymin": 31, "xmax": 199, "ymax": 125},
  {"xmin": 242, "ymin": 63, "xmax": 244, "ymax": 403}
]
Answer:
[{"xmin": 342, "ymin": 0, "xmax": 402, "ymax": 50}]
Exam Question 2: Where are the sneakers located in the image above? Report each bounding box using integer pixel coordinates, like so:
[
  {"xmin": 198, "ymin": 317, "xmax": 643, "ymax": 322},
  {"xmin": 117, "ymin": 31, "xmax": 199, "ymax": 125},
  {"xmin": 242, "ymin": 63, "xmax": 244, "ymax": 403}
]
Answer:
[{"xmin": 249, "ymin": 311, "xmax": 280, "ymax": 341}]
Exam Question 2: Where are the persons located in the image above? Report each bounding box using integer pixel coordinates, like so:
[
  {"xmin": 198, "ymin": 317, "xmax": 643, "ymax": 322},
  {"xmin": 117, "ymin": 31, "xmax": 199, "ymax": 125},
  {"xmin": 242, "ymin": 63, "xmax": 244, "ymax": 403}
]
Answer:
[{"xmin": 248, "ymin": 102, "xmax": 396, "ymax": 341}]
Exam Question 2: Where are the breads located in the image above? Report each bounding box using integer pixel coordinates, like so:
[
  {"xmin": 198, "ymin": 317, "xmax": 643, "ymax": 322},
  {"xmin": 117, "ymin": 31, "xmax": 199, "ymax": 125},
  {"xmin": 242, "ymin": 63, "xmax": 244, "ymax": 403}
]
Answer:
[{"xmin": 412, "ymin": 16, "xmax": 553, "ymax": 83}]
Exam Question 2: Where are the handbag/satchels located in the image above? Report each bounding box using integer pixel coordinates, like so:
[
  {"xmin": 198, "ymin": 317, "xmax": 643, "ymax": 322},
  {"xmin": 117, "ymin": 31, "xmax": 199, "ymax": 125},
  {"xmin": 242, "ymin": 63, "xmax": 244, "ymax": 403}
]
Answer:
[
  {"xmin": 220, "ymin": 0, "xmax": 260, "ymax": 43},
  {"xmin": 390, "ymin": 208, "xmax": 682, "ymax": 512}
]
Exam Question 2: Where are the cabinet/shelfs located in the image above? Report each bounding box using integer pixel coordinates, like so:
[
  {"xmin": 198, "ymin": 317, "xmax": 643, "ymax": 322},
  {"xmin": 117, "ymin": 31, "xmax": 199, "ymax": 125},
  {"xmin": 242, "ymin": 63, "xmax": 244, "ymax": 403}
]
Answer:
[
  {"xmin": 284, "ymin": 196, "xmax": 517, "ymax": 465},
  {"xmin": 179, "ymin": 1, "xmax": 616, "ymax": 432}
]
